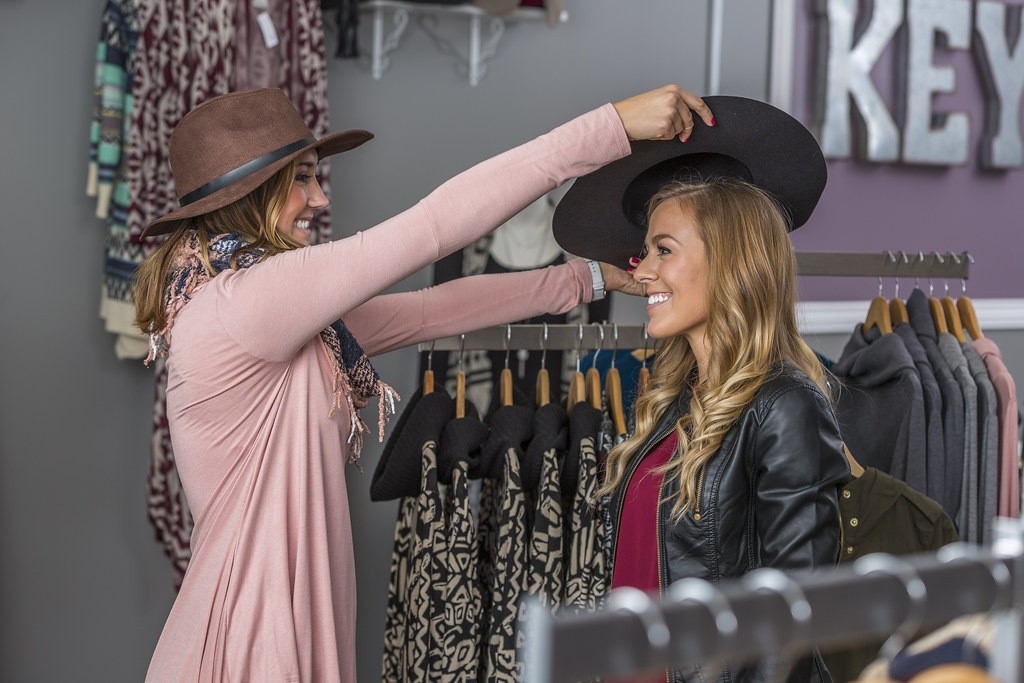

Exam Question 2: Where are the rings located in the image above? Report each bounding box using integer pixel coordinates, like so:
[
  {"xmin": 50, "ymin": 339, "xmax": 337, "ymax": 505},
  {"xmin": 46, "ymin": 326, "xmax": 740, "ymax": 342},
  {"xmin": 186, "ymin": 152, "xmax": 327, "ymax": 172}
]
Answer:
[{"xmin": 685, "ymin": 123, "xmax": 694, "ymax": 129}]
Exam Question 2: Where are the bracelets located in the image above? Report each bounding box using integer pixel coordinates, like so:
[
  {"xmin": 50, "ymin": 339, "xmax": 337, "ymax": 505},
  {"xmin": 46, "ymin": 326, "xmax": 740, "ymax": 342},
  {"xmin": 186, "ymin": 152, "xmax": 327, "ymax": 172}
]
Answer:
[{"xmin": 585, "ymin": 257, "xmax": 605, "ymax": 302}]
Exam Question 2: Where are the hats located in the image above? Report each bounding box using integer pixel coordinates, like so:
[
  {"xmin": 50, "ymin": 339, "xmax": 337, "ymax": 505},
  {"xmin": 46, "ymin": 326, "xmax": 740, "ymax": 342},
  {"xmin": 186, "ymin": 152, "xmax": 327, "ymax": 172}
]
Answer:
[
  {"xmin": 552, "ymin": 96, "xmax": 828, "ymax": 272},
  {"xmin": 139, "ymin": 87, "xmax": 373, "ymax": 240}
]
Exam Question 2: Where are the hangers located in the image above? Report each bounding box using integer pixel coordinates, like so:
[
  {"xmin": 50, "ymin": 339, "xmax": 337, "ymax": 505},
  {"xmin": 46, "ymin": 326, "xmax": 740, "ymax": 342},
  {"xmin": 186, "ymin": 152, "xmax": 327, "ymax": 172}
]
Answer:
[
  {"xmin": 597, "ymin": 526, "xmax": 1023, "ymax": 682},
  {"xmin": 423, "ymin": 249, "xmax": 981, "ymax": 429}
]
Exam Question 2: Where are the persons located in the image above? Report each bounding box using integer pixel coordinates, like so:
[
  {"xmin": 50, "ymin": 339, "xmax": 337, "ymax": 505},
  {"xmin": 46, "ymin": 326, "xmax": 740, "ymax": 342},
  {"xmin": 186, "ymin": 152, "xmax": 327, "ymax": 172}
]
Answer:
[
  {"xmin": 141, "ymin": 84, "xmax": 716, "ymax": 682},
  {"xmin": 606, "ymin": 175, "xmax": 852, "ymax": 683}
]
